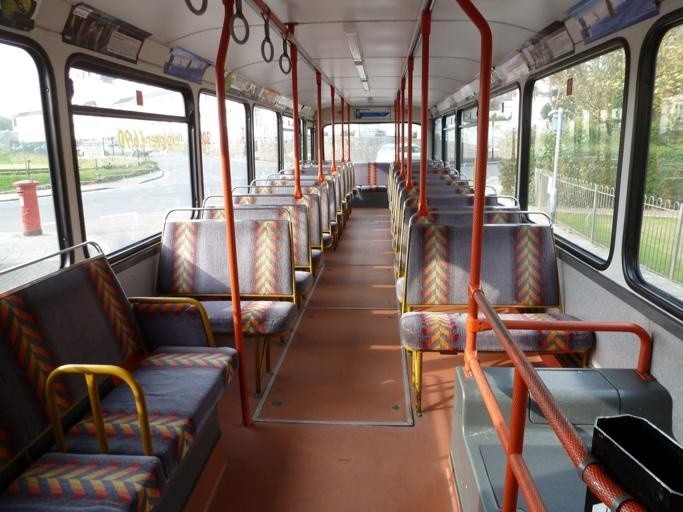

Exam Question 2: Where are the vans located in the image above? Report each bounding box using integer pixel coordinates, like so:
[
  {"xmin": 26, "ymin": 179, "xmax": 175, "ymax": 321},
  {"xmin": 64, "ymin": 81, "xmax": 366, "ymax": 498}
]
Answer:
[{"xmin": 376, "ymin": 144, "xmax": 421, "ymax": 162}]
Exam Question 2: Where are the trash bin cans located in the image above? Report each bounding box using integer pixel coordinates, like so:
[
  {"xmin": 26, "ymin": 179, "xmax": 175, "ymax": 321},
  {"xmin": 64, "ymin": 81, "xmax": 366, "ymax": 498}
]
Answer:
[{"xmin": 580, "ymin": 409, "xmax": 679, "ymax": 510}]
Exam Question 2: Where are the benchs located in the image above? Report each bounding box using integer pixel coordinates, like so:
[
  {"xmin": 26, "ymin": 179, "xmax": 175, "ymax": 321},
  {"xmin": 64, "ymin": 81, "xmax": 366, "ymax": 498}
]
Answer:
[
  {"xmin": 352, "ymin": 159, "xmax": 388, "ymax": 194},
  {"xmin": 400, "ymin": 210, "xmax": 597, "ymax": 418},
  {"xmin": 153, "ymin": 207, "xmax": 299, "ymax": 394},
  {"xmin": 387, "ymin": 157, "xmax": 521, "ymax": 308},
  {"xmin": 0, "ymin": 241, "xmax": 240, "ymax": 512},
  {"xmin": 199, "ymin": 161, "xmax": 354, "ymax": 304}
]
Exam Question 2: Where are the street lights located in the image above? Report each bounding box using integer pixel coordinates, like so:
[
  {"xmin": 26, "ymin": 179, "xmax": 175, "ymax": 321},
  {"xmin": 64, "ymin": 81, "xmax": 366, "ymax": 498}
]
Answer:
[{"xmin": 546, "ymin": 107, "xmax": 565, "ymax": 226}]
